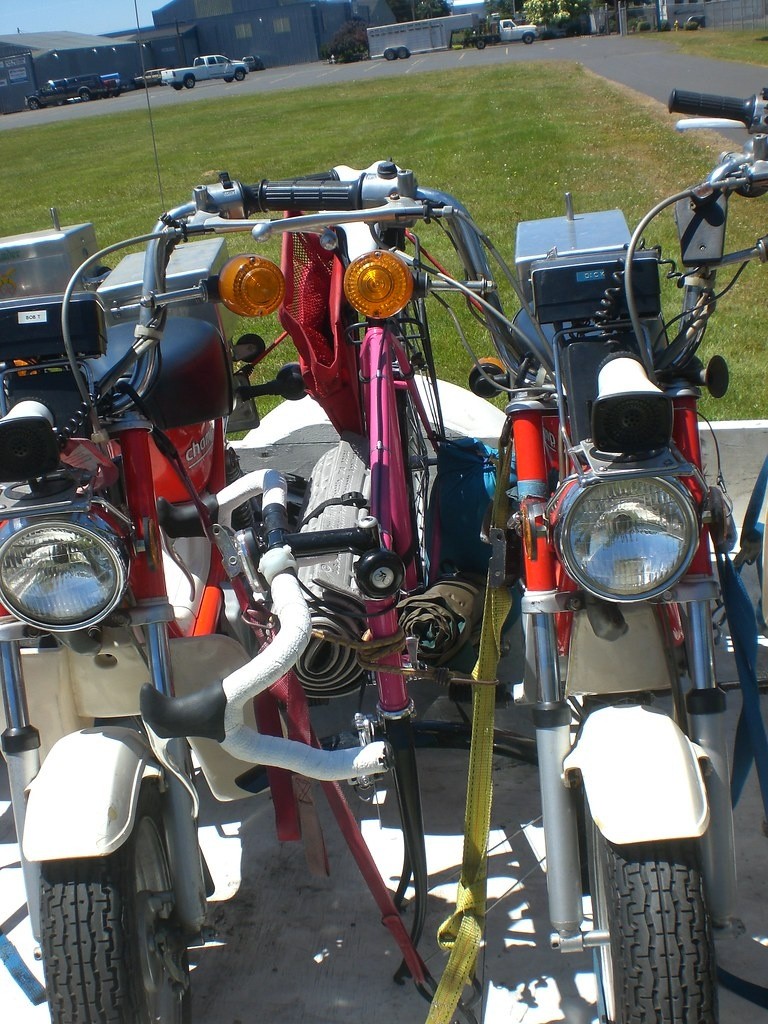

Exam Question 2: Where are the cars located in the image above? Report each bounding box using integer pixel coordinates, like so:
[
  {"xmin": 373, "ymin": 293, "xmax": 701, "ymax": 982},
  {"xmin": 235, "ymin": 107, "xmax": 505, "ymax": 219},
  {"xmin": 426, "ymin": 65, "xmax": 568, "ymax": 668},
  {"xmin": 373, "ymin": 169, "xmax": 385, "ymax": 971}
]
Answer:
[
  {"xmin": 687, "ymin": 15, "xmax": 705, "ymax": 29},
  {"xmin": 27, "ymin": 73, "xmax": 121, "ymax": 111},
  {"xmin": 134, "ymin": 68, "xmax": 167, "ymax": 89},
  {"xmin": 242, "ymin": 56, "xmax": 263, "ymax": 71}
]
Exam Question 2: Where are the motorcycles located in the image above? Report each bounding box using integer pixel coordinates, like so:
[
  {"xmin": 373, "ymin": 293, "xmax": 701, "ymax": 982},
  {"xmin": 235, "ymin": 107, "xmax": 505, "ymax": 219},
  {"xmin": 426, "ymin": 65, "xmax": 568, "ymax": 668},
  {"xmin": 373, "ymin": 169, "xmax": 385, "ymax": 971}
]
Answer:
[{"xmin": 0, "ymin": 88, "xmax": 768, "ymax": 1024}]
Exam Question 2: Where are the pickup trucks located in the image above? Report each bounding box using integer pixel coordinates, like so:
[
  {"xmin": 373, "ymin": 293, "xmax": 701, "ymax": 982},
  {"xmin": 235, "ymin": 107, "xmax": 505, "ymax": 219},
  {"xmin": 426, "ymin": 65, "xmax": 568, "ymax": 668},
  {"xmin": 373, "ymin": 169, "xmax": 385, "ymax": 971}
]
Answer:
[
  {"xmin": 161, "ymin": 55, "xmax": 249, "ymax": 90},
  {"xmin": 464, "ymin": 19, "xmax": 539, "ymax": 50}
]
